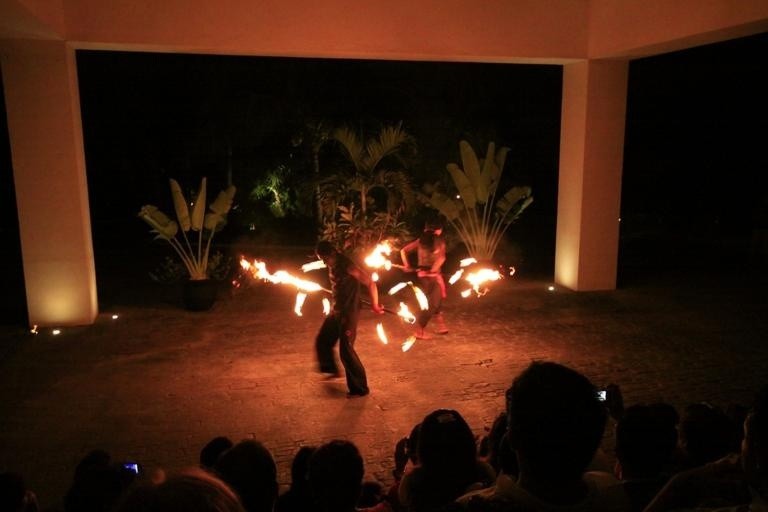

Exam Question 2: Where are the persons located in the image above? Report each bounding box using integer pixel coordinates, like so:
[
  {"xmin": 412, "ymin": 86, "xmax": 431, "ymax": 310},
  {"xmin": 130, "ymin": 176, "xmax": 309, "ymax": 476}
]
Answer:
[
  {"xmin": 315, "ymin": 240, "xmax": 385, "ymax": 399},
  {"xmin": 0, "ymin": 472, "xmax": 40, "ymax": 512},
  {"xmin": 64, "ymin": 436, "xmax": 385, "ymax": 512},
  {"xmin": 357, "ymin": 361, "xmax": 767, "ymax": 512},
  {"xmin": 400, "ymin": 220, "xmax": 447, "ymax": 340}
]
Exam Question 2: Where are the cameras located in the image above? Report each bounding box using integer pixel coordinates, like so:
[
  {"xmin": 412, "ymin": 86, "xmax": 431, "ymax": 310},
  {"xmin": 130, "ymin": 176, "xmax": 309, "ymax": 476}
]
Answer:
[
  {"xmin": 596, "ymin": 389, "xmax": 607, "ymax": 402},
  {"xmin": 123, "ymin": 462, "xmax": 141, "ymax": 476}
]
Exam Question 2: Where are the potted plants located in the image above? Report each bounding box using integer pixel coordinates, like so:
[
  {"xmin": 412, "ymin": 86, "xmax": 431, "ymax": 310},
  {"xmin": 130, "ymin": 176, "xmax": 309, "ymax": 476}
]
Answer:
[
  {"xmin": 136, "ymin": 173, "xmax": 240, "ymax": 311},
  {"xmin": 416, "ymin": 138, "xmax": 530, "ymax": 292}
]
